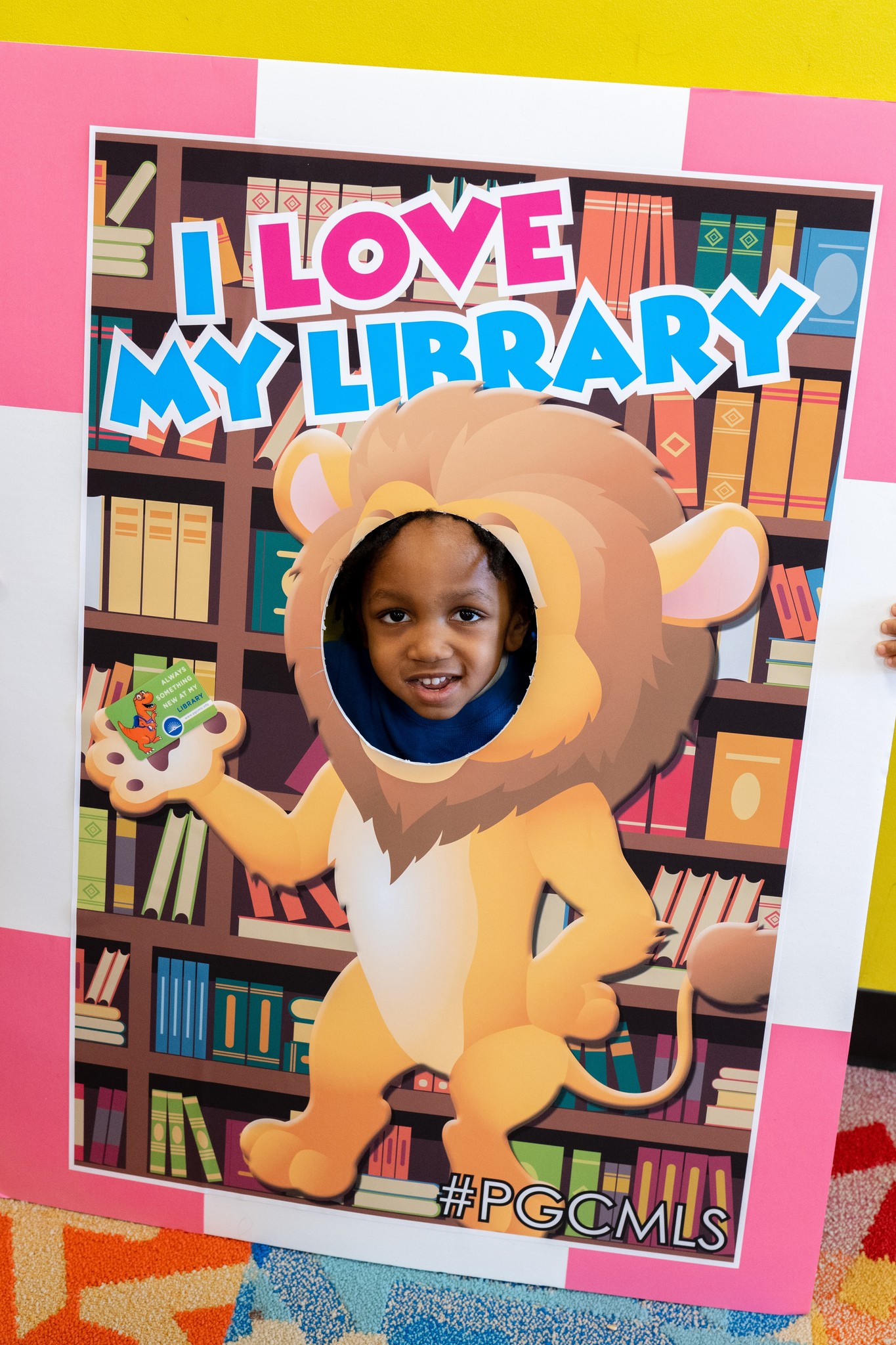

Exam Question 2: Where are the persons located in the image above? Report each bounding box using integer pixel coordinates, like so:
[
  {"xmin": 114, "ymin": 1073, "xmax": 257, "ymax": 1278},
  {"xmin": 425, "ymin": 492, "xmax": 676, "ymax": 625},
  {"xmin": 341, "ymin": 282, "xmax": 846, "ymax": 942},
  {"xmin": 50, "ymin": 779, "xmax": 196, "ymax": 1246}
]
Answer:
[{"xmin": 325, "ymin": 510, "xmax": 896, "ymax": 763}]
